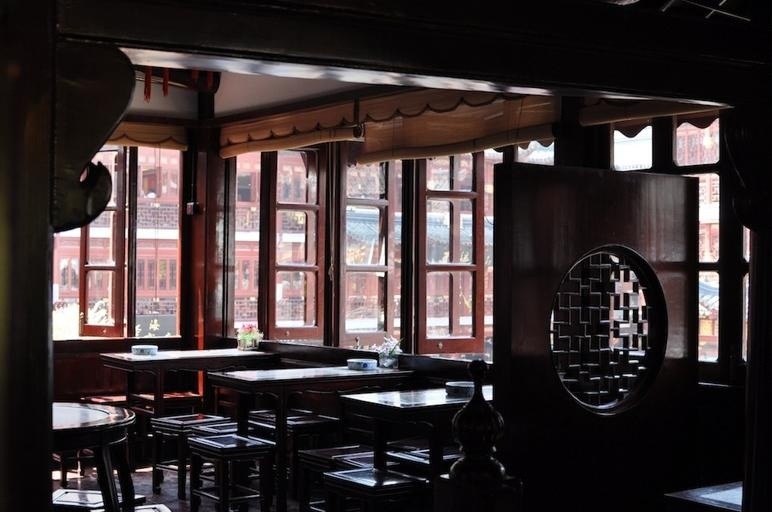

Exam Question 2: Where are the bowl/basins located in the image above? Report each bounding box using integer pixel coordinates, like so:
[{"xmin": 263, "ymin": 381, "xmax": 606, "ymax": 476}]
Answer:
[
  {"xmin": 347, "ymin": 358, "xmax": 377, "ymax": 370},
  {"xmin": 132, "ymin": 345, "xmax": 158, "ymax": 355},
  {"xmin": 445, "ymin": 382, "xmax": 475, "ymax": 397}
]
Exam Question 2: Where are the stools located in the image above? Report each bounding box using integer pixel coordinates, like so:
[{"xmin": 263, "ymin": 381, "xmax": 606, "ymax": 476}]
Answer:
[{"xmin": 53, "ymin": 389, "xmax": 460, "ymax": 510}]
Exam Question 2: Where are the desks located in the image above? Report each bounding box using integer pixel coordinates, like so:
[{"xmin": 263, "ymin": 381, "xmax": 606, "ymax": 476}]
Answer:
[
  {"xmin": 101, "ymin": 348, "xmax": 287, "ymax": 495},
  {"xmin": 338, "ymin": 379, "xmax": 492, "ymax": 505},
  {"xmin": 206, "ymin": 361, "xmax": 413, "ymax": 498}
]
